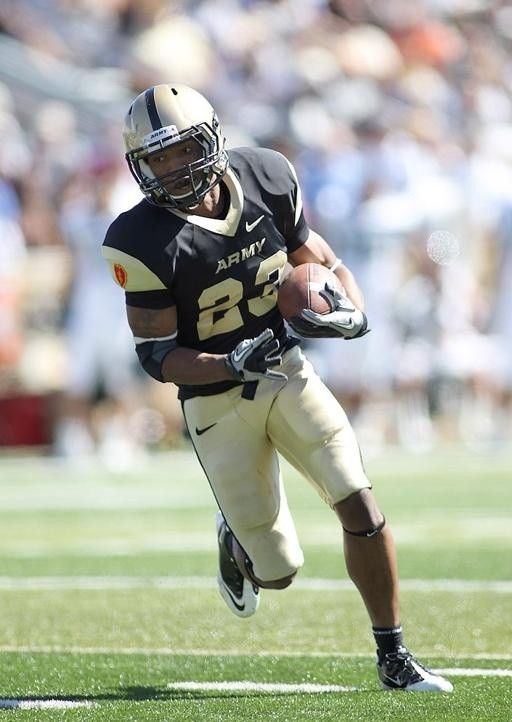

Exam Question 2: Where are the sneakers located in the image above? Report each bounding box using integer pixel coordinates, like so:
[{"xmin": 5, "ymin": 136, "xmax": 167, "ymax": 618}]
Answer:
[
  {"xmin": 376, "ymin": 643, "xmax": 454, "ymax": 693},
  {"xmin": 216, "ymin": 509, "xmax": 260, "ymax": 618}
]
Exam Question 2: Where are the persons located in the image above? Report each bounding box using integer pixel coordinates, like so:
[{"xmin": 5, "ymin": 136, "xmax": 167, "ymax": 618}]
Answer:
[{"xmin": 101, "ymin": 81, "xmax": 453, "ymax": 695}]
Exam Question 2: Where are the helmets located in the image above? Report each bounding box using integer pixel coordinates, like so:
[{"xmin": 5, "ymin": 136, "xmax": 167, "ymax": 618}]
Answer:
[{"xmin": 121, "ymin": 84, "xmax": 226, "ymax": 203}]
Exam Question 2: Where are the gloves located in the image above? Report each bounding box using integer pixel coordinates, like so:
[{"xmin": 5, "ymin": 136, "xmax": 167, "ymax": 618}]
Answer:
[
  {"xmin": 224, "ymin": 327, "xmax": 288, "ymax": 382},
  {"xmin": 288, "ymin": 281, "xmax": 372, "ymax": 340}
]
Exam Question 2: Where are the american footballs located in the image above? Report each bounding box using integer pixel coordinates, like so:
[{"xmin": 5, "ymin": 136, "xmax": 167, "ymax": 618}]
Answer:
[{"xmin": 279, "ymin": 262, "xmax": 350, "ymax": 323}]
